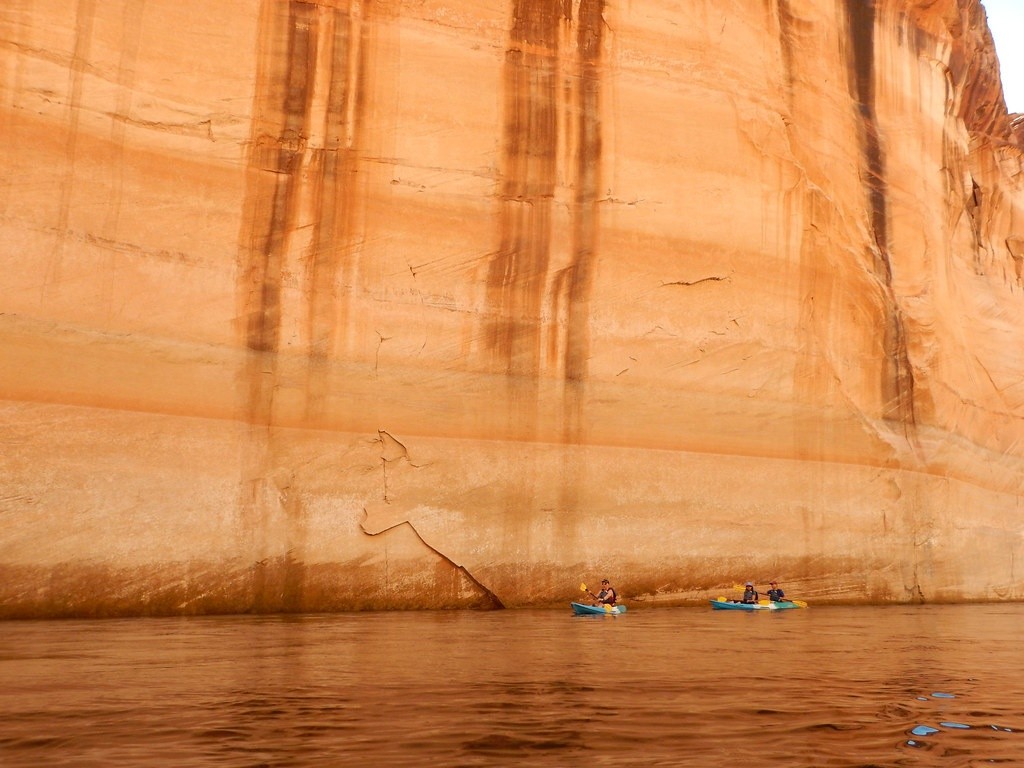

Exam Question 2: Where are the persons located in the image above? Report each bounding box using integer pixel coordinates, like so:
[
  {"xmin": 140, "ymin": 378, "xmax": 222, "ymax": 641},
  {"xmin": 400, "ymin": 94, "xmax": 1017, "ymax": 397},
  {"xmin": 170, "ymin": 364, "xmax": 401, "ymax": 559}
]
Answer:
[
  {"xmin": 590, "ymin": 580, "xmax": 616, "ymax": 608},
  {"xmin": 730, "ymin": 582, "xmax": 758, "ymax": 604},
  {"xmin": 767, "ymin": 580, "xmax": 784, "ymax": 602}
]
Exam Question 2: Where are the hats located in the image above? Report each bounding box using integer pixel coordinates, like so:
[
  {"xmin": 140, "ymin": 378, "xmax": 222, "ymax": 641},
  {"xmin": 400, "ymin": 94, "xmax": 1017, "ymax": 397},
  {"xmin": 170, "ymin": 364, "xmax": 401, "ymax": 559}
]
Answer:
[
  {"xmin": 746, "ymin": 582, "xmax": 753, "ymax": 587},
  {"xmin": 769, "ymin": 580, "xmax": 777, "ymax": 585}
]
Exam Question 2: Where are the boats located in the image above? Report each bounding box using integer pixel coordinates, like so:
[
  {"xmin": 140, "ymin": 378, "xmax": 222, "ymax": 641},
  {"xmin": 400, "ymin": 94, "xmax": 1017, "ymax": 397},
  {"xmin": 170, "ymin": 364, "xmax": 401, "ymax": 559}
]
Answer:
[
  {"xmin": 570, "ymin": 602, "xmax": 627, "ymax": 613},
  {"xmin": 709, "ymin": 594, "xmax": 808, "ymax": 610}
]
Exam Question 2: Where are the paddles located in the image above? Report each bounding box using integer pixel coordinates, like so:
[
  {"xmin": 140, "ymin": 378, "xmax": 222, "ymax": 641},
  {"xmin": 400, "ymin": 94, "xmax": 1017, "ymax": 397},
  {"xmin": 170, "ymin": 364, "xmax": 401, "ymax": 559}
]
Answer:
[
  {"xmin": 735, "ymin": 585, "xmax": 808, "ymax": 608},
  {"xmin": 717, "ymin": 596, "xmax": 770, "ymax": 607},
  {"xmin": 579, "ymin": 582, "xmax": 612, "ymax": 612}
]
